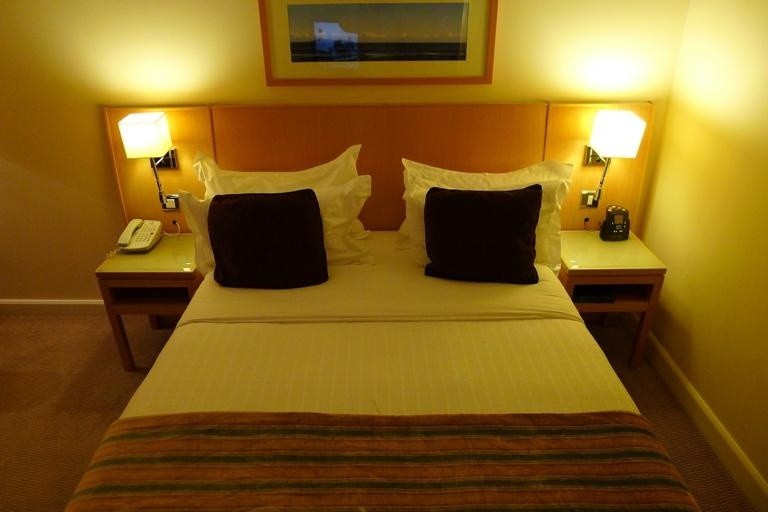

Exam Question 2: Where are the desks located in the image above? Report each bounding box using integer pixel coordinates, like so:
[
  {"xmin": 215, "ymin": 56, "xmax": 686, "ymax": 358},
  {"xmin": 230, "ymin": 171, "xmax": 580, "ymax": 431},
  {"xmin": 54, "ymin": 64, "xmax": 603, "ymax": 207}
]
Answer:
[
  {"xmin": 557, "ymin": 229, "xmax": 668, "ymax": 367},
  {"xmin": 95, "ymin": 232, "xmax": 204, "ymax": 372}
]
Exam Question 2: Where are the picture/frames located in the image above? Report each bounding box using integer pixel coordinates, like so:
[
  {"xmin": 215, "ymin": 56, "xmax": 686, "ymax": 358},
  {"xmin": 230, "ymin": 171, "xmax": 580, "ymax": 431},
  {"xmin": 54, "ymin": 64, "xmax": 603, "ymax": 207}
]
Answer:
[{"xmin": 257, "ymin": 0, "xmax": 498, "ymax": 87}]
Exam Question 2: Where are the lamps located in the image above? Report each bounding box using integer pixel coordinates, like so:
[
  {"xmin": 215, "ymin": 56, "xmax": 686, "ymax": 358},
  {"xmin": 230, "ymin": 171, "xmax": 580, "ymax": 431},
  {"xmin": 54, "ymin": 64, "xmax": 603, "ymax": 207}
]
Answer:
[
  {"xmin": 117, "ymin": 113, "xmax": 179, "ymax": 204},
  {"xmin": 579, "ymin": 109, "xmax": 646, "ymax": 209}
]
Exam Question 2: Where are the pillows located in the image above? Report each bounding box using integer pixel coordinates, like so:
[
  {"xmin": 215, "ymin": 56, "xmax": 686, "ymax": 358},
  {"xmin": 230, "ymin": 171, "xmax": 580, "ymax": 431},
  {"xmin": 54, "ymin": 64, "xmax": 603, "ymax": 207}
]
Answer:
[
  {"xmin": 402, "ymin": 171, "xmax": 569, "ymax": 277},
  {"xmin": 400, "ymin": 159, "xmax": 570, "ymax": 245},
  {"xmin": 192, "ymin": 144, "xmax": 370, "ymax": 240},
  {"xmin": 208, "ymin": 187, "xmax": 326, "ymax": 289},
  {"xmin": 424, "ymin": 185, "xmax": 542, "ymax": 285},
  {"xmin": 179, "ymin": 174, "xmax": 375, "ymax": 274}
]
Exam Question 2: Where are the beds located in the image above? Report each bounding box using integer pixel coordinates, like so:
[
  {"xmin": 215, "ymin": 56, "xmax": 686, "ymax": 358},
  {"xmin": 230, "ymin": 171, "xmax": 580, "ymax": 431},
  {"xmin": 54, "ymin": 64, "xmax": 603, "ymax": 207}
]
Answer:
[{"xmin": 62, "ymin": 227, "xmax": 702, "ymax": 511}]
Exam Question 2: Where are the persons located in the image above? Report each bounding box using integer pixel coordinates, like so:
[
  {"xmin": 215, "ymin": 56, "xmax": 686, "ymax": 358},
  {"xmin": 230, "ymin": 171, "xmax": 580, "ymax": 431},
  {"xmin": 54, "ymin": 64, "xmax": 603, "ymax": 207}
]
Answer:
[
  {"xmin": 344, "ymin": 37, "xmax": 355, "ymax": 49},
  {"xmin": 316, "ymin": 28, "xmax": 326, "ymax": 46}
]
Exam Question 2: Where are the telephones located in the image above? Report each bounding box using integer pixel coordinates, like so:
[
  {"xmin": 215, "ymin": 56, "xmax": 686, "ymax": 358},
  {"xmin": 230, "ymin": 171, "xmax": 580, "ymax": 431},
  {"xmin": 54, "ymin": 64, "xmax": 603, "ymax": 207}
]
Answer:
[{"xmin": 118, "ymin": 218, "xmax": 164, "ymax": 252}]
